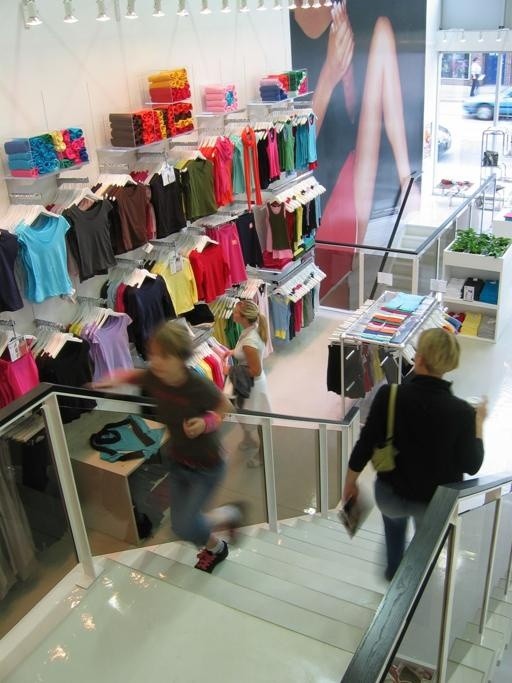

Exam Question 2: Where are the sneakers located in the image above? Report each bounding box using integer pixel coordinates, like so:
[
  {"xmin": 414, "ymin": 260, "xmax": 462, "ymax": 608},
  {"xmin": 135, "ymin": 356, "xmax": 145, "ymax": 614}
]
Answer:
[{"xmin": 193, "ymin": 538, "xmax": 229, "ymax": 574}]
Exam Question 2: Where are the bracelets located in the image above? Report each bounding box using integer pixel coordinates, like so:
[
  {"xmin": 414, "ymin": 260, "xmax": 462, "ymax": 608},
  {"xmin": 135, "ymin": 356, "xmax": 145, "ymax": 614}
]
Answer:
[
  {"xmin": 209, "ymin": 410, "xmax": 224, "ymax": 427},
  {"xmin": 204, "ymin": 413, "xmax": 223, "ymax": 435}
]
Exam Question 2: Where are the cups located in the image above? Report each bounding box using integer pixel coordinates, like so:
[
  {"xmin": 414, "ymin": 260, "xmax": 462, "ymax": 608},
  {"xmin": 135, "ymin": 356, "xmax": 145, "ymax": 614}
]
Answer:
[{"xmin": 467, "ymin": 395, "xmax": 482, "ymax": 409}]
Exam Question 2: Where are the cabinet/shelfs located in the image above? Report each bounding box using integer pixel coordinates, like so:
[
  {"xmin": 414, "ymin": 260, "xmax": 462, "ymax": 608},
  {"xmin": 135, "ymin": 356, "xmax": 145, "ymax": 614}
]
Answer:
[
  {"xmin": 60, "ymin": 409, "xmax": 173, "ymax": 549},
  {"xmin": 442, "ymin": 236, "xmax": 512, "ymax": 345}
]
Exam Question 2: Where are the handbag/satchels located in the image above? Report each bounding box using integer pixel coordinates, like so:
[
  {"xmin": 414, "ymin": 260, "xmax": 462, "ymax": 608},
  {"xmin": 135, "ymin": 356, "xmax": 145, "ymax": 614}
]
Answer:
[{"xmin": 370, "ymin": 382, "xmax": 401, "ymax": 474}]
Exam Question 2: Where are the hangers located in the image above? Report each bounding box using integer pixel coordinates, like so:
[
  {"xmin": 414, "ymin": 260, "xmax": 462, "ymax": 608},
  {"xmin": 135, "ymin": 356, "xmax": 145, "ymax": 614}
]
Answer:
[
  {"xmin": 330, "ymin": 294, "xmax": 446, "ymax": 365},
  {"xmin": 0, "ymin": 101, "xmax": 327, "ymax": 358}
]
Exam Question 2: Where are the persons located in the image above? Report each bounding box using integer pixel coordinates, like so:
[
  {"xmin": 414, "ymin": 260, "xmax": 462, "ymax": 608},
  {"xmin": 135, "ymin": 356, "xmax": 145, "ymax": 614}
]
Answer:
[
  {"xmin": 469, "ymin": 56, "xmax": 480, "ymax": 97},
  {"xmin": 82, "ymin": 318, "xmax": 247, "ymax": 578},
  {"xmin": 289, "ymin": 0, "xmax": 419, "ymax": 310},
  {"xmin": 219, "ymin": 298, "xmax": 276, "ymax": 469},
  {"xmin": 338, "ymin": 325, "xmax": 490, "ymax": 586}
]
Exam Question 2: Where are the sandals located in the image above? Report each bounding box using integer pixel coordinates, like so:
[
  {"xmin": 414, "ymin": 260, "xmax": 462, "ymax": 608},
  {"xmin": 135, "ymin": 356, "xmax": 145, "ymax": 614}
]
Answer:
[{"xmin": 236, "ymin": 438, "xmax": 264, "ymax": 468}]
